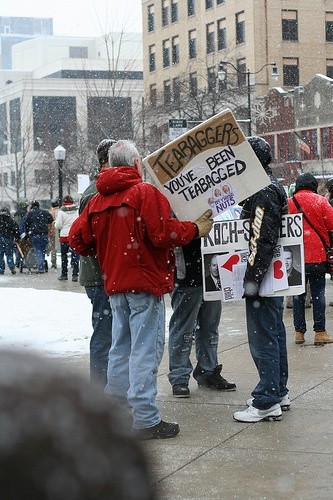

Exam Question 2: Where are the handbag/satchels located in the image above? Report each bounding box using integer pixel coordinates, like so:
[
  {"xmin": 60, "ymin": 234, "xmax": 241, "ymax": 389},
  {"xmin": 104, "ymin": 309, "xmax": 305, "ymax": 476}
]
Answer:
[{"xmin": 325, "ymin": 245, "xmax": 333, "ymax": 272}]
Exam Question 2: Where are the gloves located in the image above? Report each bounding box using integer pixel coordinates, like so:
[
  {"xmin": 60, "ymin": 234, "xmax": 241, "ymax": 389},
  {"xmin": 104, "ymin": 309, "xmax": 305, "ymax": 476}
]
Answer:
[
  {"xmin": 193, "ymin": 209, "xmax": 213, "ymax": 239},
  {"xmin": 242, "ymin": 284, "xmax": 259, "ymax": 299}
]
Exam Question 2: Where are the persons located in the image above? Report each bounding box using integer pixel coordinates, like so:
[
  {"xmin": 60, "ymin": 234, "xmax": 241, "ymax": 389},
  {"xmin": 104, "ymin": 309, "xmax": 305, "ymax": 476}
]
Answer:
[
  {"xmin": 71, "ymin": 137, "xmax": 114, "ymax": 396},
  {"xmin": 231, "ymin": 136, "xmax": 298, "ymax": 426},
  {"xmin": 165, "ymin": 208, "xmax": 235, "ymax": 398},
  {"xmin": 11, "ymin": 204, "xmax": 31, "ymax": 268},
  {"xmin": 283, "ymin": 172, "xmax": 332, "ymax": 346},
  {"xmin": 46, "ymin": 201, "xmax": 67, "ymax": 268},
  {"xmin": 0, "ymin": 207, "xmax": 20, "ymax": 275},
  {"xmin": 24, "ymin": 200, "xmax": 55, "ymax": 276},
  {"xmin": 281, "ymin": 245, "xmax": 302, "ymax": 286},
  {"xmin": 54, "ymin": 194, "xmax": 82, "ymax": 282},
  {"xmin": 322, "ymin": 178, "xmax": 333, "ymax": 203},
  {"xmin": 205, "ymin": 254, "xmax": 222, "ymax": 292},
  {"xmin": 68, "ymin": 139, "xmax": 214, "ymax": 442}
]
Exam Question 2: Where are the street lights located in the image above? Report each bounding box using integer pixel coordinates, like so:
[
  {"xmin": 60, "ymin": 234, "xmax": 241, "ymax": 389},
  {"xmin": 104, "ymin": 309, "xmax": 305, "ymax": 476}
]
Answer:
[
  {"xmin": 53, "ymin": 140, "xmax": 67, "ymax": 238},
  {"xmin": 218, "ymin": 60, "xmax": 279, "ymax": 137}
]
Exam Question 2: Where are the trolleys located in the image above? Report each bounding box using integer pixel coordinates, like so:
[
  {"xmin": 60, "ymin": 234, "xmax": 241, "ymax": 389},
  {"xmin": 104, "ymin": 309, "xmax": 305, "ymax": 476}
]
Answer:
[{"xmin": 12, "ymin": 240, "xmax": 48, "ymax": 273}]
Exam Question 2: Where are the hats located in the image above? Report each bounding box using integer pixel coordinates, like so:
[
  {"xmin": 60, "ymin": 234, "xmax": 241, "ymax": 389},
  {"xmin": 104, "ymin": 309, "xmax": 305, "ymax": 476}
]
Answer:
[
  {"xmin": 248, "ymin": 136, "xmax": 272, "ymax": 166},
  {"xmin": 295, "ymin": 173, "xmax": 318, "ymax": 192}
]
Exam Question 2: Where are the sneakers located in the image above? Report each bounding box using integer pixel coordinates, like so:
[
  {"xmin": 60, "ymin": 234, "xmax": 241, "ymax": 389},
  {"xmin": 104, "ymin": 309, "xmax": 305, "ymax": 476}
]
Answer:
[
  {"xmin": 314, "ymin": 331, "xmax": 333, "ymax": 345},
  {"xmin": 58, "ymin": 275, "xmax": 68, "ymax": 280},
  {"xmin": 193, "ymin": 364, "xmax": 236, "ymax": 391},
  {"xmin": 232, "ymin": 394, "xmax": 292, "ymax": 423},
  {"xmin": 72, "ymin": 274, "xmax": 78, "ymax": 282},
  {"xmin": 295, "ymin": 331, "xmax": 305, "ymax": 344},
  {"xmin": 133, "ymin": 421, "xmax": 180, "ymax": 441},
  {"xmin": 173, "ymin": 383, "xmax": 191, "ymax": 397}
]
